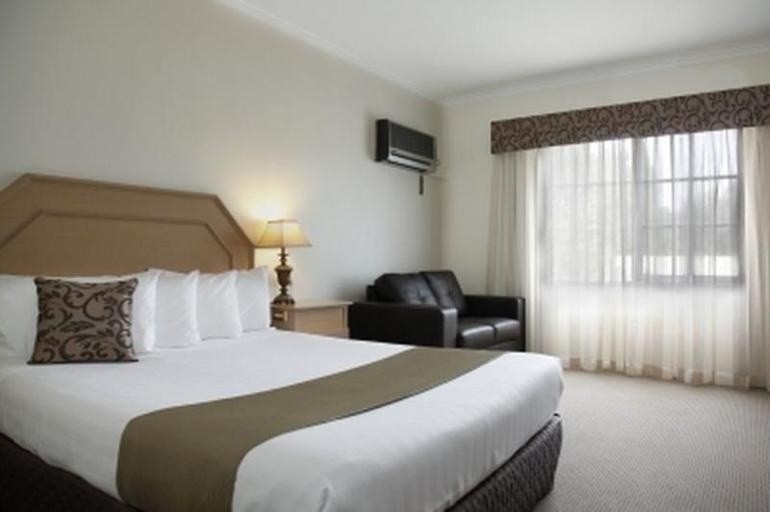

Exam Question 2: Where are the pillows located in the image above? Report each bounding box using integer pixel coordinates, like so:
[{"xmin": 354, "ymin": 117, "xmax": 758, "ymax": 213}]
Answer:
[{"xmin": 1, "ymin": 266, "xmax": 273, "ymax": 364}]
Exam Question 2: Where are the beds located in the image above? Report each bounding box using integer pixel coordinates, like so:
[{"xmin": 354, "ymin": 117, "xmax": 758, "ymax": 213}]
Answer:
[{"xmin": 1, "ymin": 174, "xmax": 562, "ymax": 512}]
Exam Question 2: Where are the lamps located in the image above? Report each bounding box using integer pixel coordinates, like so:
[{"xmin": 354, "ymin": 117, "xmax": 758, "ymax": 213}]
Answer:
[{"xmin": 252, "ymin": 219, "xmax": 314, "ymax": 306}]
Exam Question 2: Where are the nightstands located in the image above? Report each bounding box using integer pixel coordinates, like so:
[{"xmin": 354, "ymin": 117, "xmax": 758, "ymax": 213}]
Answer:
[{"xmin": 269, "ymin": 301, "xmax": 354, "ymax": 340}]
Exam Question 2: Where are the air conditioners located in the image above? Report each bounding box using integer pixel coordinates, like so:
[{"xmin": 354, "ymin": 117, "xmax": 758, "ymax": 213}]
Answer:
[{"xmin": 376, "ymin": 118, "xmax": 438, "ymax": 174}]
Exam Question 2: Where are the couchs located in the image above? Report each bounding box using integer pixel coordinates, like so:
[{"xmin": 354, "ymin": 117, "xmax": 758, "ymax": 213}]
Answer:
[{"xmin": 348, "ymin": 270, "xmax": 525, "ymax": 353}]
